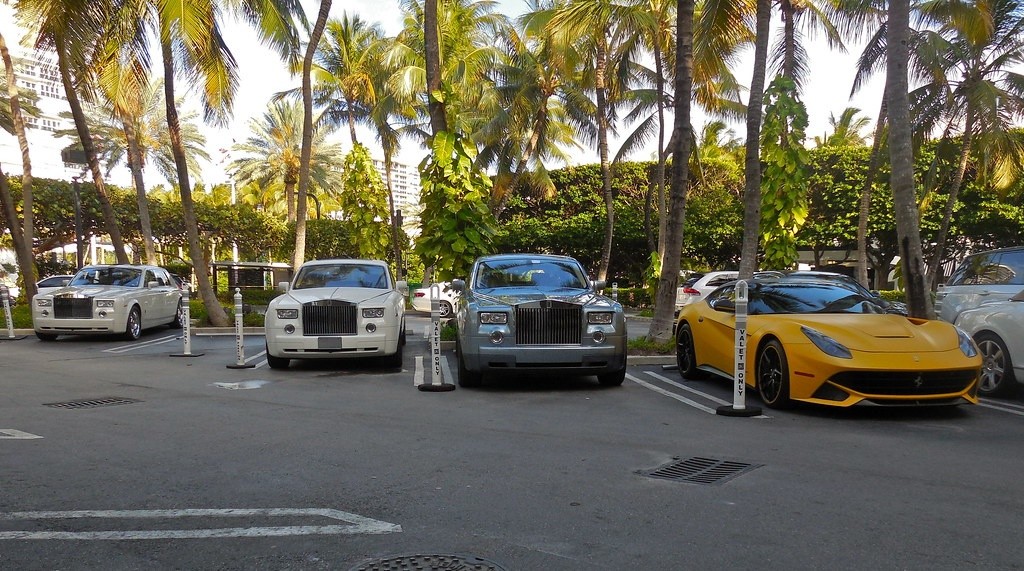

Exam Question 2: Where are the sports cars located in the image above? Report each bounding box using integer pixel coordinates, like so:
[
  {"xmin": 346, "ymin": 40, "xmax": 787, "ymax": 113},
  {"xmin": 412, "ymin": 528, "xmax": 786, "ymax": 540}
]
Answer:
[{"xmin": 675, "ymin": 278, "xmax": 983, "ymax": 410}]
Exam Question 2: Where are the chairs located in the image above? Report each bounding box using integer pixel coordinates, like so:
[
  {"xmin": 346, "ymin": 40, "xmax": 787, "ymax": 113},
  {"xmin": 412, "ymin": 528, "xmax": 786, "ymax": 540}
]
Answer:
[{"xmin": 530, "ymin": 272, "xmax": 551, "ymax": 285}]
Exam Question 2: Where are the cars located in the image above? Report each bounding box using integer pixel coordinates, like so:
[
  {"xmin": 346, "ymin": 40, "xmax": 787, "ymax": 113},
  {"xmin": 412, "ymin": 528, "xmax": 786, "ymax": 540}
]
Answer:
[
  {"xmin": 34, "ymin": 275, "xmax": 99, "ymax": 297},
  {"xmin": 412, "ymin": 278, "xmax": 465, "ymax": 317},
  {"xmin": 677, "ymin": 270, "xmax": 785, "ymax": 314},
  {"xmin": 265, "ymin": 259, "xmax": 406, "ymax": 373},
  {"xmin": 32, "ymin": 264, "xmax": 183, "ymax": 340},
  {"xmin": 938, "ymin": 246, "xmax": 1024, "ymax": 397},
  {"xmin": 452, "ymin": 253, "xmax": 627, "ymax": 387}
]
